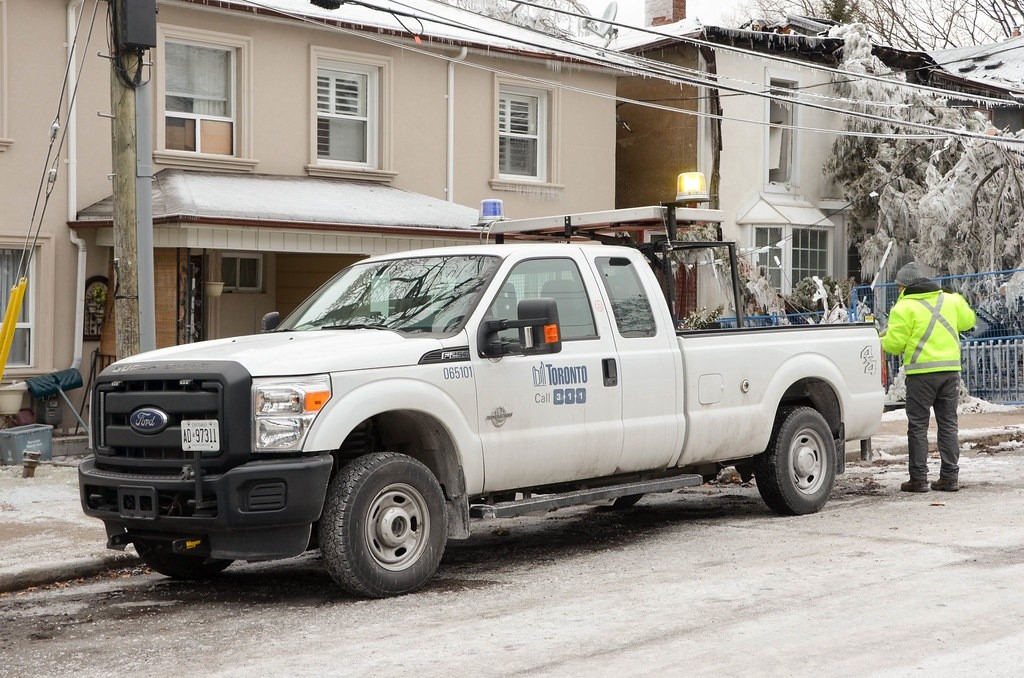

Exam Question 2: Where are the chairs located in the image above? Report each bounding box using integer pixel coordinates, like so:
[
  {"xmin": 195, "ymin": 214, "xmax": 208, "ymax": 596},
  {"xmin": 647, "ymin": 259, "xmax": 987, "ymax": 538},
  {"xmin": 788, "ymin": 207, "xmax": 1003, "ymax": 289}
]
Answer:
[{"xmin": 540, "ymin": 279, "xmax": 590, "ymax": 338}]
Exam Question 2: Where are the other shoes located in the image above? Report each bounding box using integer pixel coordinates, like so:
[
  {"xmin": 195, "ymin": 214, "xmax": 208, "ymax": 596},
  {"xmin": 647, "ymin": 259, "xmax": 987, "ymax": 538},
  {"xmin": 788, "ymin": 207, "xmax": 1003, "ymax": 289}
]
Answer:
[
  {"xmin": 931, "ymin": 478, "xmax": 959, "ymax": 491},
  {"xmin": 901, "ymin": 479, "xmax": 929, "ymax": 491}
]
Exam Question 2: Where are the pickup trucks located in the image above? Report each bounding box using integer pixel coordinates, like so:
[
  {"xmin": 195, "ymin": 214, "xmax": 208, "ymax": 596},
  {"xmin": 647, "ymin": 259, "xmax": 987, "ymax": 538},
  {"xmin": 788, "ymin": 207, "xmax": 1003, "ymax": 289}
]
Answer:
[{"xmin": 77, "ymin": 239, "xmax": 886, "ymax": 600}]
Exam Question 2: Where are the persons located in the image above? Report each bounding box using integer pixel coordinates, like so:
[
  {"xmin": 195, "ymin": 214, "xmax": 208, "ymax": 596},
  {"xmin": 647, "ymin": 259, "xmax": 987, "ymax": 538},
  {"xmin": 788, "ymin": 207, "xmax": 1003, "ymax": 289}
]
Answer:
[{"xmin": 880, "ymin": 261, "xmax": 977, "ymax": 493}]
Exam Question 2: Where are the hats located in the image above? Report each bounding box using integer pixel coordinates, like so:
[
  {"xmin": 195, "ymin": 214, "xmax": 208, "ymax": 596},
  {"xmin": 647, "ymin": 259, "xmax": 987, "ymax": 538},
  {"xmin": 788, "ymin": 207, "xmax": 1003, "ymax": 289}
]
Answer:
[{"xmin": 894, "ymin": 262, "xmax": 922, "ymax": 287}]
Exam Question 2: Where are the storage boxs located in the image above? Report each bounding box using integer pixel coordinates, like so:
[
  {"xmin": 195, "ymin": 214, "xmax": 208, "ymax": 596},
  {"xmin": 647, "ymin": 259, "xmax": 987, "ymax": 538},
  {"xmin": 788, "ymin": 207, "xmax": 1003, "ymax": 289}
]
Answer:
[{"xmin": 0, "ymin": 424, "xmax": 54, "ymax": 465}]
